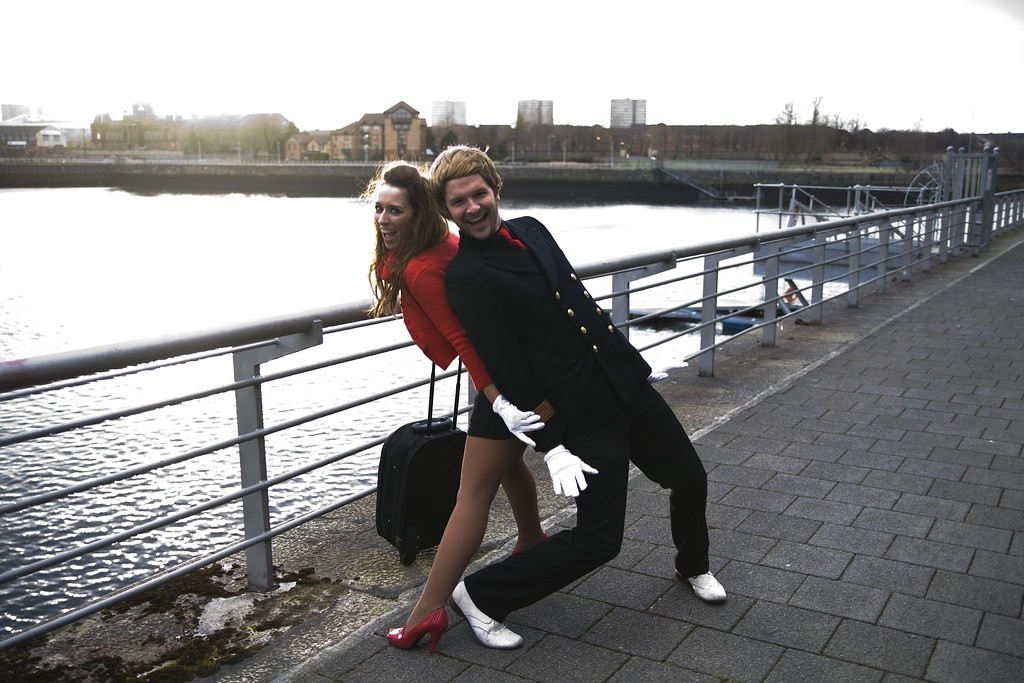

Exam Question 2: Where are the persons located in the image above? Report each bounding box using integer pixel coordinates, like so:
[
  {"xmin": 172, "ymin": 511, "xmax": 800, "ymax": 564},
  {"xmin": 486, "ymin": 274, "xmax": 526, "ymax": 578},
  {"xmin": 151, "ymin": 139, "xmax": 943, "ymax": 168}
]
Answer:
[
  {"xmin": 428, "ymin": 142, "xmax": 727, "ymax": 649},
  {"xmin": 366, "ymin": 163, "xmax": 547, "ymax": 651}
]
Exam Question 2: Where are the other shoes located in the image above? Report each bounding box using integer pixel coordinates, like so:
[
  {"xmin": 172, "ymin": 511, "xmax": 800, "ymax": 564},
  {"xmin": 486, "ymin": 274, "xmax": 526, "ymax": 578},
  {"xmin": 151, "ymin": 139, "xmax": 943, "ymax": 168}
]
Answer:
[
  {"xmin": 675, "ymin": 567, "xmax": 726, "ymax": 601},
  {"xmin": 449, "ymin": 580, "xmax": 523, "ymax": 650}
]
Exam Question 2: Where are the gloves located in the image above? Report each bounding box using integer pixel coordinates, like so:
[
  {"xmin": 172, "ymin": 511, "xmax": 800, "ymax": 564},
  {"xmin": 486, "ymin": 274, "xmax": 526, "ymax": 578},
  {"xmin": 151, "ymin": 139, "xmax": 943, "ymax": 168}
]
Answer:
[
  {"xmin": 492, "ymin": 394, "xmax": 545, "ymax": 447},
  {"xmin": 543, "ymin": 445, "xmax": 599, "ymax": 497}
]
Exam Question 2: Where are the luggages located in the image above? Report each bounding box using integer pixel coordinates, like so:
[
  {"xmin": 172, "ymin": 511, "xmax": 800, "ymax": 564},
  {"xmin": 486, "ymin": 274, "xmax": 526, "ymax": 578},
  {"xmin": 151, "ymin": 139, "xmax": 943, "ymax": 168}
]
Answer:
[{"xmin": 375, "ymin": 354, "xmax": 467, "ymax": 567}]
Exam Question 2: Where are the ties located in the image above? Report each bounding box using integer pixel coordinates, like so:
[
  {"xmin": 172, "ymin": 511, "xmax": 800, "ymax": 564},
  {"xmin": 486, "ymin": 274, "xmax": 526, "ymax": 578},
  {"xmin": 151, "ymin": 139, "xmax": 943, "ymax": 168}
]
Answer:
[{"xmin": 497, "ymin": 227, "xmax": 529, "ymax": 253}]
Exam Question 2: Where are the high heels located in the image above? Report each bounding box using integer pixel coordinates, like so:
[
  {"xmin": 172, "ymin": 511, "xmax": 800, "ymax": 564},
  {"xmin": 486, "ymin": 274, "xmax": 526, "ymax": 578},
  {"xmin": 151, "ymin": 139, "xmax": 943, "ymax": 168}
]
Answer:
[
  {"xmin": 386, "ymin": 608, "xmax": 448, "ymax": 654},
  {"xmin": 511, "ymin": 531, "xmax": 548, "ymax": 556}
]
